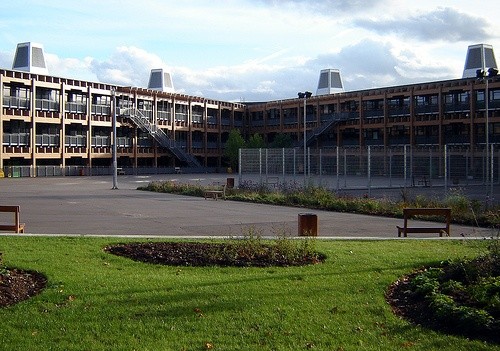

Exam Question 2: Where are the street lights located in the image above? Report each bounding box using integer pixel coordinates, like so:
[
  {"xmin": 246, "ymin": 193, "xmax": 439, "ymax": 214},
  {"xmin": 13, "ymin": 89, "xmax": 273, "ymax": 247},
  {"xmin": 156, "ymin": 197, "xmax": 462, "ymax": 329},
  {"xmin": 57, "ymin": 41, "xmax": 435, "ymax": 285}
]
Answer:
[
  {"xmin": 474, "ymin": 67, "xmax": 498, "ymax": 201},
  {"xmin": 298, "ymin": 91, "xmax": 313, "ymax": 192},
  {"xmin": 112, "ymin": 87, "xmax": 119, "ymax": 190}
]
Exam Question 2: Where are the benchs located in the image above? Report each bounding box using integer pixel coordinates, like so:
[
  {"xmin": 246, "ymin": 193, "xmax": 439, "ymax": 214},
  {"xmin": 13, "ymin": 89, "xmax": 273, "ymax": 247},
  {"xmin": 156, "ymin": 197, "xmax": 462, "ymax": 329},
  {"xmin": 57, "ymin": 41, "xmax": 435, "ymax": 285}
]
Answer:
[
  {"xmin": 0, "ymin": 205, "xmax": 25, "ymax": 233},
  {"xmin": 204, "ymin": 190, "xmax": 224, "ymax": 201},
  {"xmin": 117, "ymin": 168, "xmax": 125, "ymax": 175},
  {"xmin": 396, "ymin": 208, "xmax": 452, "ymax": 237},
  {"xmin": 263, "ymin": 176, "xmax": 279, "ymax": 189},
  {"xmin": 175, "ymin": 167, "xmax": 182, "ymax": 174}
]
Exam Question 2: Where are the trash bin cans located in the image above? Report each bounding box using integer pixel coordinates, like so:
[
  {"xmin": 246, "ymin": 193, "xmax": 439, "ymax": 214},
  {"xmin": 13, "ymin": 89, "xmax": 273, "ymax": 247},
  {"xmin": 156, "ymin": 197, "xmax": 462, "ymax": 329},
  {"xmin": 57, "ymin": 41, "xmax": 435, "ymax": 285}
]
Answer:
[
  {"xmin": 297, "ymin": 212, "xmax": 317, "ymax": 238},
  {"xmin": 226, "ymin": 177, "xmax": 235, "ymax": 190}
]
